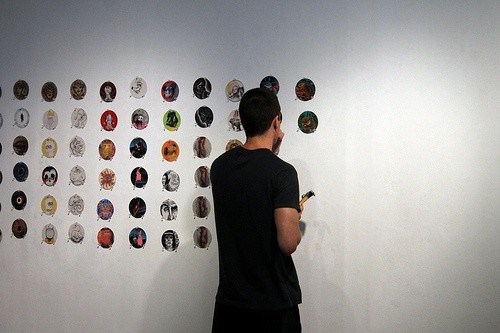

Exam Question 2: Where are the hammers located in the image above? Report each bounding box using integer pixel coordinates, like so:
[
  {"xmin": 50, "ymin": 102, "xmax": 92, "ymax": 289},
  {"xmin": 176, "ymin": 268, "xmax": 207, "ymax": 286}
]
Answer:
[{"xmin": 299, "ymin": 190, "xmax": 316, "ymax": 207}]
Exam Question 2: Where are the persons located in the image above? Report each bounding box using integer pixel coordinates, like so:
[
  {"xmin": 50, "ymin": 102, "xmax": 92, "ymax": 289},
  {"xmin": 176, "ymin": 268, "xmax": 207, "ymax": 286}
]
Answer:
[{"xmin": 210, "ymin": 88, "xmax": 305, "ymax": 333}]
